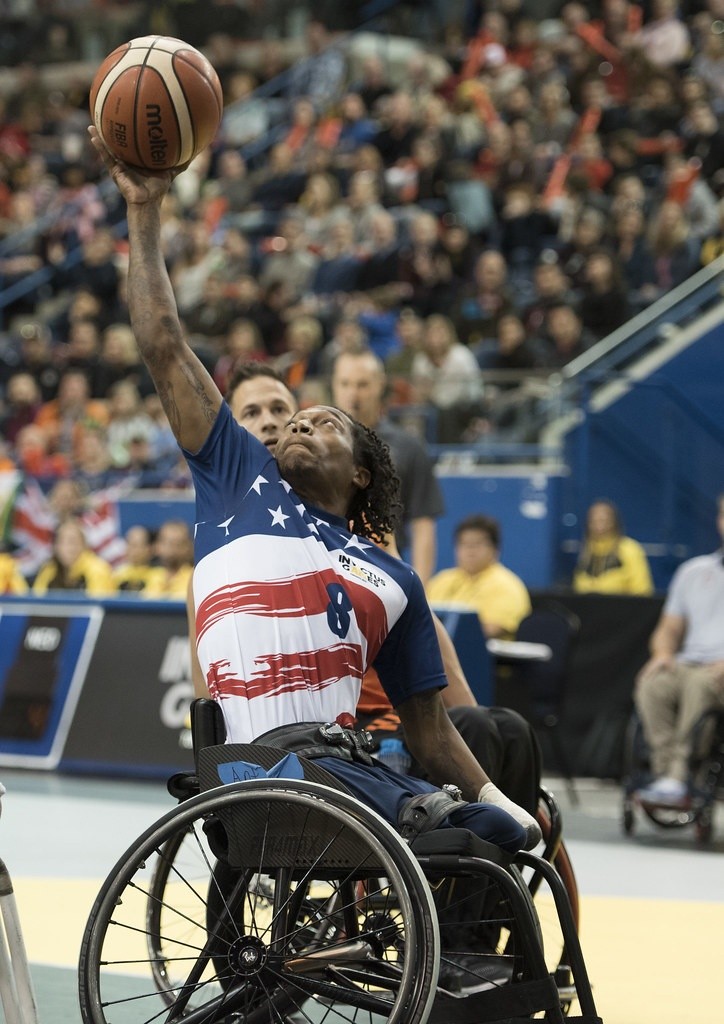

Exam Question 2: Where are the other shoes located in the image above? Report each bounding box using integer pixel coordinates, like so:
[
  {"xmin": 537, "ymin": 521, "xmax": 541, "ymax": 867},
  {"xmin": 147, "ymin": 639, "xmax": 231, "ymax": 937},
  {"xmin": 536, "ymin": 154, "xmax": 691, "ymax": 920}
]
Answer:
[
  {"xmin": 396, "ymin": 944, "xmax": 514, "ymax": 999},
  {"xmin": 633, "ymin": 777, "xmax": 691, "ymax": 807}
]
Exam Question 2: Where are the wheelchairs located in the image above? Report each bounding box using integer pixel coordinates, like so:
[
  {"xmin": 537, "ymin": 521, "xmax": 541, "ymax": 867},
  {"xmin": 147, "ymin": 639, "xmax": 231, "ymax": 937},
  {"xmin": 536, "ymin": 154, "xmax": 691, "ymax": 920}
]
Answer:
[
  {"xmin": 621, "ymin": 700, "xmax": 724, "ymax": 849},
  {"xmin": 80, "ymin": 698, "xmax": 606, "ymax": 1024}
]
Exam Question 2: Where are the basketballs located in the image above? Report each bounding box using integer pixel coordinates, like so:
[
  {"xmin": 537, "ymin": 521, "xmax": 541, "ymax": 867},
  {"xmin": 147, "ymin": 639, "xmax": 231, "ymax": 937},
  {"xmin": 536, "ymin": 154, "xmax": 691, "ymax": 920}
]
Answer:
[{"xmin": 87, "ymin": 32, "xmax": 228, "ymax": 176}]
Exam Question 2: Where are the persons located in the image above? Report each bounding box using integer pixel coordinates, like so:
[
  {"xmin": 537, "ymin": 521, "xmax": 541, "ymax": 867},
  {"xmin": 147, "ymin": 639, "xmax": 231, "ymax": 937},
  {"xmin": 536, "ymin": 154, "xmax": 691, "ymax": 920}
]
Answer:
[
  {"xmin": 0, "ymin": 0, "xmax": 724, "ymax": 504},
  {"xmin": 188, "ymin": 364, "xmax": 544, "ymax": 951},
  {"xmin": 633, "ymin": 494, "xmax": 724, "ymax": 805},
  {"xmin": 87, "ymin": 125, "xmax": 545, "ymax": 997},
  {"xmin": 571, "ymin": 503, "xmax": 655, "ymax": 596},
  {"xmin": 0, "ymin": 519, "xmax": 533, "ymax": 637}
]
